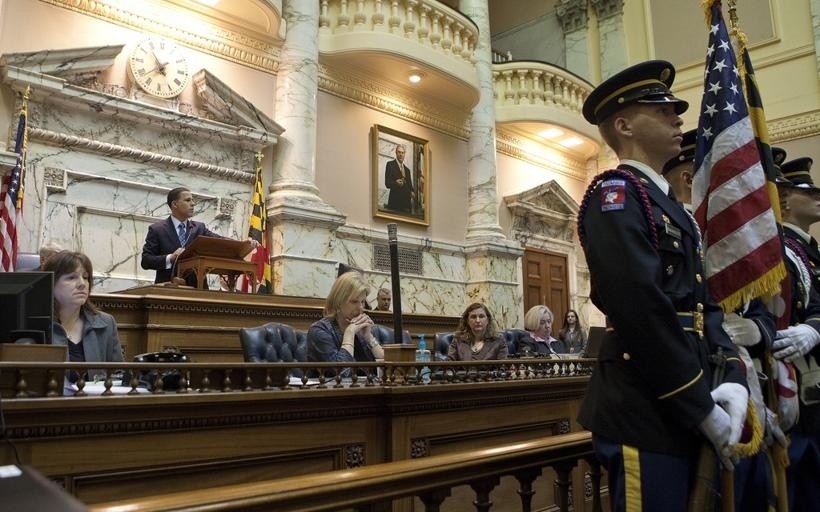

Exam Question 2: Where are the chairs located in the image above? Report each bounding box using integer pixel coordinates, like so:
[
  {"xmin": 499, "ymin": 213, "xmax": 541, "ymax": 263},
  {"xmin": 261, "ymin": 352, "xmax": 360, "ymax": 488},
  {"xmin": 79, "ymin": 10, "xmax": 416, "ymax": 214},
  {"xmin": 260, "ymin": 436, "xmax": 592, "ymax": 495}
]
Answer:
[
  {"xmin": 433, "ymin": 330, "xmax": 453, "ymax": 358},
  {"xmin": 369, "ymin": 323, "xmax": 414, "ymax": 347},
  {"xmin": 505, "ymin": 326, "xmax": 532, "ymax": 360},
  {"xmin": 238, "ymin": 321, "xmax": 310, "ymax": 376}
]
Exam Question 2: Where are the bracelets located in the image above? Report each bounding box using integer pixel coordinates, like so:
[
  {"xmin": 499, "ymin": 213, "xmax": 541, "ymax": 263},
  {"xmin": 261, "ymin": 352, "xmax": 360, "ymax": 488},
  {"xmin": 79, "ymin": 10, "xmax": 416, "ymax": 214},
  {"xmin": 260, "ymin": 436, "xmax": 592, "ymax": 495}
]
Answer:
[
  {"xmin": 366, "ymin": 337, "xmax": 380, "ymax": 350},
  {"xmin": 341, "ymin": 342, "xmax": 355, "ymax": 348}
]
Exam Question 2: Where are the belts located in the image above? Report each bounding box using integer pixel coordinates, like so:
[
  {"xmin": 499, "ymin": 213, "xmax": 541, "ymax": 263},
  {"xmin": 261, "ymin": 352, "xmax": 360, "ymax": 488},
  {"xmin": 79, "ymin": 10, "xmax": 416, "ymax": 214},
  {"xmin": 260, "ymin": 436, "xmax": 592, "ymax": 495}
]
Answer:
[{"xmin": 605, "ymin": 312, "xmax": 704, "ymax": 334}]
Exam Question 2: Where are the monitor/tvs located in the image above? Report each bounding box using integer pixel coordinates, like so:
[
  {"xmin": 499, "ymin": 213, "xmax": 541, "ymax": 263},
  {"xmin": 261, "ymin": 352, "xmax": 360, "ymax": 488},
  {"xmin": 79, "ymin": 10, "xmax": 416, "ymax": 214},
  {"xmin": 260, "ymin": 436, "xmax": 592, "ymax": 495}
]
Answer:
[{"xmin": 0, "ymin": 271, "xmax": 55, "ymax": 344}]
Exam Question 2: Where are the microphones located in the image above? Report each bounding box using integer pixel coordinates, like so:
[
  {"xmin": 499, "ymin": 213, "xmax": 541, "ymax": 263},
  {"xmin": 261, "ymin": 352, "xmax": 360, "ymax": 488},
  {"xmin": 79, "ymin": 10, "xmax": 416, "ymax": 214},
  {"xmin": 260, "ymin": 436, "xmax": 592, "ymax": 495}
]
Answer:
[{"xmin": 164, "ymin": 224, "xmax": 193, "ymax": 286}]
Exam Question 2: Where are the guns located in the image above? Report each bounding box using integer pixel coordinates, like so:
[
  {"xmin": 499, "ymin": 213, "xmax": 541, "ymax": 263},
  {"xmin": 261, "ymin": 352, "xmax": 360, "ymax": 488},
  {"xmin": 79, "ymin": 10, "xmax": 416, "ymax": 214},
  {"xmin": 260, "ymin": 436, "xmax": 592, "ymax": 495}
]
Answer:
[{"xmin": 688, "ymin": 348, "xmax": 727, "ymax": 512}]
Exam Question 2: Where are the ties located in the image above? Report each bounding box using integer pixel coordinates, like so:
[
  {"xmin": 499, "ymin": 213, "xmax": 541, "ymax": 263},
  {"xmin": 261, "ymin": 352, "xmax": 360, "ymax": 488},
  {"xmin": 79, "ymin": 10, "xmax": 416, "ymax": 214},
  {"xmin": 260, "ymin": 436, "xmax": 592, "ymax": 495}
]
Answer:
[
  {"xmin": 400, "ymin": 164, "xmax": 406, "ymax": 177},
  {"xmin": 810, "ymin": 236, "xmax": 818, "ymax": 251},
  {"xmin": 179, "ymin": 223, "xmax": 186, "ymax": 246},
  {"xmin": 668, "ymin": 186, "xmax": 677, "ymax": 205}
]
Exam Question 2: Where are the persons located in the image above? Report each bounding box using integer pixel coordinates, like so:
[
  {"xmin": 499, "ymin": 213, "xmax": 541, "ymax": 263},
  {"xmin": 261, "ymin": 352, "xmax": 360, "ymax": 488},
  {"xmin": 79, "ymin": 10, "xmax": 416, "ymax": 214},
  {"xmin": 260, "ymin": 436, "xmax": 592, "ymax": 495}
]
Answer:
[
  {"xmin": 140, "ymin": 188, "xmax": 261, "ymax": 291},
  {"xmin": 304, "ymin": 271, "xmax": 385, "ymax": 381},
  {"xmin": 384, "ymin": 145, "xmax": 416, "ymax": 215},
  {"xmin": 42, "ymin": 251, "xmax": 128, "ymax": 383},
  {"xmin": 517, "ymin": 305, "xmax": 565, "ymax": 369},
  {"xmin": 438, "ymin": 302, "xmax": 509, "ymax": 372},
  {"xmin": 558, "ymin": 310, "xmax": 587, "ymax": 359},
  {"xmin": 30, "ymin": 246, "xmax": 59, "ymax": 271},
  {"xmin": 372, "ymin": 288, "xmax": 392, "ymax": 312}
]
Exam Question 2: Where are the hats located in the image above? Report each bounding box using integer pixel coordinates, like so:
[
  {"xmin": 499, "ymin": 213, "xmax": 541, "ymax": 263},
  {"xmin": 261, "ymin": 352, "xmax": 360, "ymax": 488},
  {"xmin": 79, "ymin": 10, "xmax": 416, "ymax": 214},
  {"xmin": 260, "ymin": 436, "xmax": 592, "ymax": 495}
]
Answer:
[
  {"xmin": 780, "ymin": 157, "xmax": 820, "ymax": 189},
  {"xmin": 772, "ymin": 147, "xmax": 793, "ymax": 186},
  {"xmin": 582, "ymin": 59, "xmax": 688, "ymax": 126},
  {"xmin": 663, "ymin": 129, "xmax": 697, "ymax": 175}
]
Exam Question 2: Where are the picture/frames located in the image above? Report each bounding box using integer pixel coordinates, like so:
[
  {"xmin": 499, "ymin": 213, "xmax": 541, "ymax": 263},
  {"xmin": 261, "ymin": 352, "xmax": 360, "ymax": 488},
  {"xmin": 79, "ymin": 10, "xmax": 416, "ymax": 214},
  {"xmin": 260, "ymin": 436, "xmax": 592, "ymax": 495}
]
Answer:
[{"xmin": 372, "ymin": 123, "xmax": 430, "ymax": 226}]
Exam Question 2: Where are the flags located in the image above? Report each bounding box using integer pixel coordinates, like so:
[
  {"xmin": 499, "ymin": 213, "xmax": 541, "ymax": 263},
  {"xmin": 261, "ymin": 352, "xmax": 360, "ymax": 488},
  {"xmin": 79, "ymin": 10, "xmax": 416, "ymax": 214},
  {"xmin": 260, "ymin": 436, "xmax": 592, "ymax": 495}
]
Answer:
[
  {"xmin": 240, "ymin": 165, "xmax": 272, "ymax": 293},
  {"xmin": 0, "ymin": 109, "xmax": 27, "ymax": 272}
]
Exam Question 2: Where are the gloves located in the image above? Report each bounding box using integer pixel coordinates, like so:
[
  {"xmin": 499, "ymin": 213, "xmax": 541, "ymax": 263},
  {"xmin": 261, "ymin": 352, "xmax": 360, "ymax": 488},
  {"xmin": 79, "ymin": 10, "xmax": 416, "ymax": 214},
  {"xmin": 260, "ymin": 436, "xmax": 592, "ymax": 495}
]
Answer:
[
  {"xmin": 710, "ymin": 382, "xmax": 748, "ymax": 457},
  {"xmin": 698, "ymin": 404, "xmax": 740, "ymax": 472},
  {"xmin": 762, "ymin": 406, "xmax": 789, "ymax": 449},
  {"xmin": 722, "ymin": 313, "xmax": 762, "ymax": 346},
  {"xmin": 771, "ymin": 324, "xmax": 820, "ymax": 363}
]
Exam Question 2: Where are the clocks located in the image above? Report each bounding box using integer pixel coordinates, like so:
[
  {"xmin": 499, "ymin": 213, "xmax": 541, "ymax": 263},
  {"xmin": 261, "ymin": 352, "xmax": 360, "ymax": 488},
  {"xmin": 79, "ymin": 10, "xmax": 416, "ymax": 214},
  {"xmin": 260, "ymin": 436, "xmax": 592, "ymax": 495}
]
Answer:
[{"xmin": 131, "ymin": 37, "xmax": 191, "ymax": 100}]
